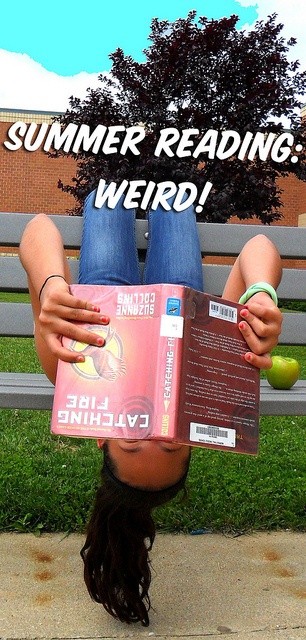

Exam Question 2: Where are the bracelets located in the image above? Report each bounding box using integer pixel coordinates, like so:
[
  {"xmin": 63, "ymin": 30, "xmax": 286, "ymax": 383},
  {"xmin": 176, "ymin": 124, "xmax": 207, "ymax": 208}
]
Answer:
[
  {"xmin": 237, "ymin": 281, "xmax": 278, "ymax": 310},
  {"xmin": 37, "ymin": 274, "xmax": 65, "ymax": 301}
]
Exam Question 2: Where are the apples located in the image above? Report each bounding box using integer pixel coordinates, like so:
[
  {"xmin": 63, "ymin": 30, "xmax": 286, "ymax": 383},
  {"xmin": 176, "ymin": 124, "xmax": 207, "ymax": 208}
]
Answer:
[{"xmin": 267, "ymin": 356, "xmax": 299, "ymax": 389}]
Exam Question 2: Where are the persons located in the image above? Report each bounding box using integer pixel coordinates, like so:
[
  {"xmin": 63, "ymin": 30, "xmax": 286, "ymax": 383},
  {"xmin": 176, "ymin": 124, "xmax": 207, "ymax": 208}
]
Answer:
[{"xmin": 17, "ymin": 184, "xmax": 286, "ymax": 630}]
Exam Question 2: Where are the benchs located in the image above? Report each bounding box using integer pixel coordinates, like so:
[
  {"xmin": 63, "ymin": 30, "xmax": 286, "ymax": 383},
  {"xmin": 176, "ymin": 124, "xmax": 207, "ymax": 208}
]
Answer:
[{"xmin": 0, "ymin": 212, "xmax": 305, "ymax": 417}]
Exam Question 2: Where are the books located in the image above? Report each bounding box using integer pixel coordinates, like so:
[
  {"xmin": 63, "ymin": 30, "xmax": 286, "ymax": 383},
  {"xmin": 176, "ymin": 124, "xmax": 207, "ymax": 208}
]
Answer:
[{"xmin": 50, "ymin": 284, "xmax": 261, "ymax": 457}]
